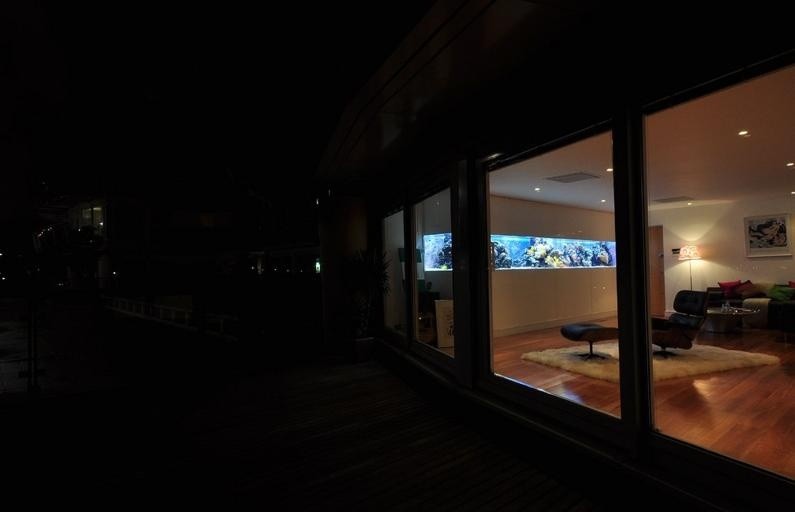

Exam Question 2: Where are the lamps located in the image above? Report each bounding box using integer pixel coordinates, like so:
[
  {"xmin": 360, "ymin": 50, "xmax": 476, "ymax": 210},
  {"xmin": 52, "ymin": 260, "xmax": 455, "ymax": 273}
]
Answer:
[{"xmin": 678, "ymin": 245, "xmax": 703, "ymax": 290}]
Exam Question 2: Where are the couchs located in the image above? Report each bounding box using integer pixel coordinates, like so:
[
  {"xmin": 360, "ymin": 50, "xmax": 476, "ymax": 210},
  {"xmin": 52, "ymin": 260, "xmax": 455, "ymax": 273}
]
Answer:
[{"xmin": 707, "ymin": 280, "xmax": 794, "ymax": 328}]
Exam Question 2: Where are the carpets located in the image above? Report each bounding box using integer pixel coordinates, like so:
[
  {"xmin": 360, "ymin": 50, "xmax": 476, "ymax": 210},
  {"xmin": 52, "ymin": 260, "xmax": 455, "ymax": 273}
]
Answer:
[{"xmin": 522, "ymin": 344, "xmax": 782, "ymax": 387}]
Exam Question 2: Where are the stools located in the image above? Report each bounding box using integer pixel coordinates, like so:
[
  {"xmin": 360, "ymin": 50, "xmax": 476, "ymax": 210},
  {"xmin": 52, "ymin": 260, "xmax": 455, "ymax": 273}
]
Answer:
[{"xmin": 560, "ymin": 322, "xmax": 621, "ymax": 360}]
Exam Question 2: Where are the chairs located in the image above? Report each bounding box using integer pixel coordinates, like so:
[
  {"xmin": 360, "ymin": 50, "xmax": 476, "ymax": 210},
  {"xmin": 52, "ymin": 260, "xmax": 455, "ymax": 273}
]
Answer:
[{"xmin": 651, "ymin": 289, "xmax": 711, "ymax": 359}]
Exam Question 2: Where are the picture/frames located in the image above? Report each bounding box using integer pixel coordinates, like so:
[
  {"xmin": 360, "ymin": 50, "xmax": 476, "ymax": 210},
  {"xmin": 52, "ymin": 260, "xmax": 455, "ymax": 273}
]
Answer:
[{"xmin": 744, "ymin": 213, "xmax": 792, "ymax": 258}]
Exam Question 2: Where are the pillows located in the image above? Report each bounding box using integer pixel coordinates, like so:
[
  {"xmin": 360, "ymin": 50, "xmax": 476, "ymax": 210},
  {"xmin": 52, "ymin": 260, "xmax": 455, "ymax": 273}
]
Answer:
[{"xmin": 718, "ymin": 278, "xmax": 795, "ymax": 302}]
